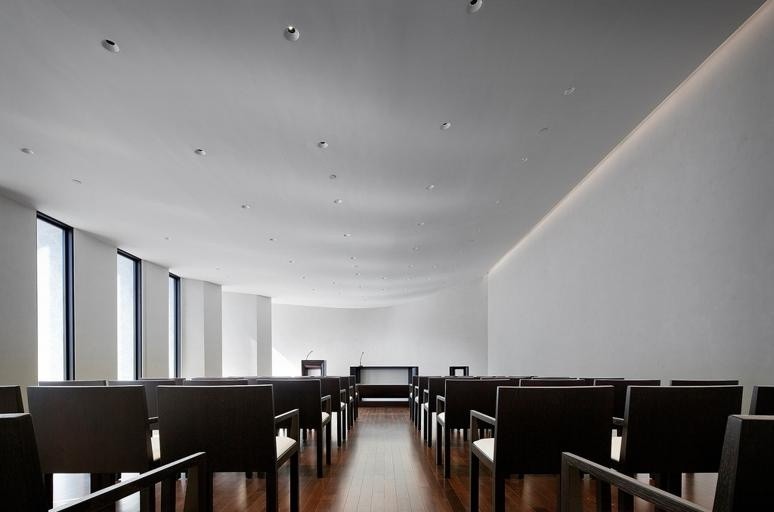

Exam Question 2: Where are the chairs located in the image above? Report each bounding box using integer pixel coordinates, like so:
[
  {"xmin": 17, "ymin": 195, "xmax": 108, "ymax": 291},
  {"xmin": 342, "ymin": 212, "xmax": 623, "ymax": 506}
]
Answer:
[
  {"xmin": 1, "ymin": 411, "xmax": 214, "ymax": 511},
  {"xmin": 1, "ymin": 384, "xmax": 25, "ymax": 414},
  {"xmin": 466, "ymin": 384, "xmax": 616, "ymax": 512},
  {"xmin": 37, "ymin": 376, "xmax": 360, "ymax": 480},
  {"xmin": 747, "ymin": 385, "xmax": 774, "ymax": 417},
  {"xmin": 26, "ymin": 383, "xmax": 159, "ymax": 511},
  {"xmin": 556, "ymin": 413, "xmax": 774, "ymax": 511},
  {"xmin": 610, "ymin": 383, "xmax": 746, "ymax": 512},
  {"xmin": 408, "ymin": 374, "xmax": 737, "ymax": 481},
  {"xmin": 155, "ymin": 383, "xmax": 300, "ymax": 511}
]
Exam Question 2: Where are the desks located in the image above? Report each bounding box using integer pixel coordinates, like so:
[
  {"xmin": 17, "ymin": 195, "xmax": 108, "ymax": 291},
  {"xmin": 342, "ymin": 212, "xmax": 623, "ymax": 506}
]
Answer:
[{"xmin": 350, "ymin": 366, "xmax": 419, "ymax": 402}]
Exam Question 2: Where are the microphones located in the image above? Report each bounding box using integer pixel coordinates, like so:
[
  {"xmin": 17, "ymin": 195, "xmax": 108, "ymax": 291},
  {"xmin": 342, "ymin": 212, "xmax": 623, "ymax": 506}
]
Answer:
[
  {"xmin": 358, "ymin": 351, "xmax": 364, "ymax": 367},
  {"xmin": 305, "ymin": 350, "xmax": 314, "ymax": 360}
]
